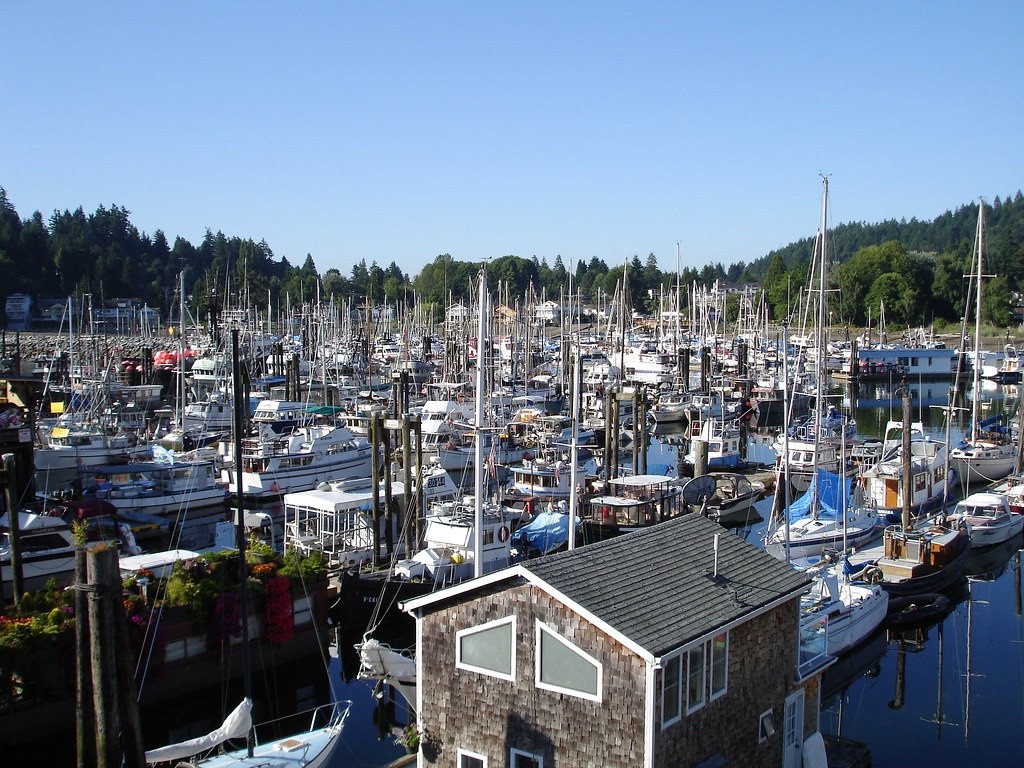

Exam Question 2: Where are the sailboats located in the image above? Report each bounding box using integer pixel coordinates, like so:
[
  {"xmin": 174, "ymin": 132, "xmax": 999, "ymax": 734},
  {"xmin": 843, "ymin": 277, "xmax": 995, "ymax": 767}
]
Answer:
[{"xmin": 0, "ymin": 172, "xmax": 1024, "ymax": 767}]
